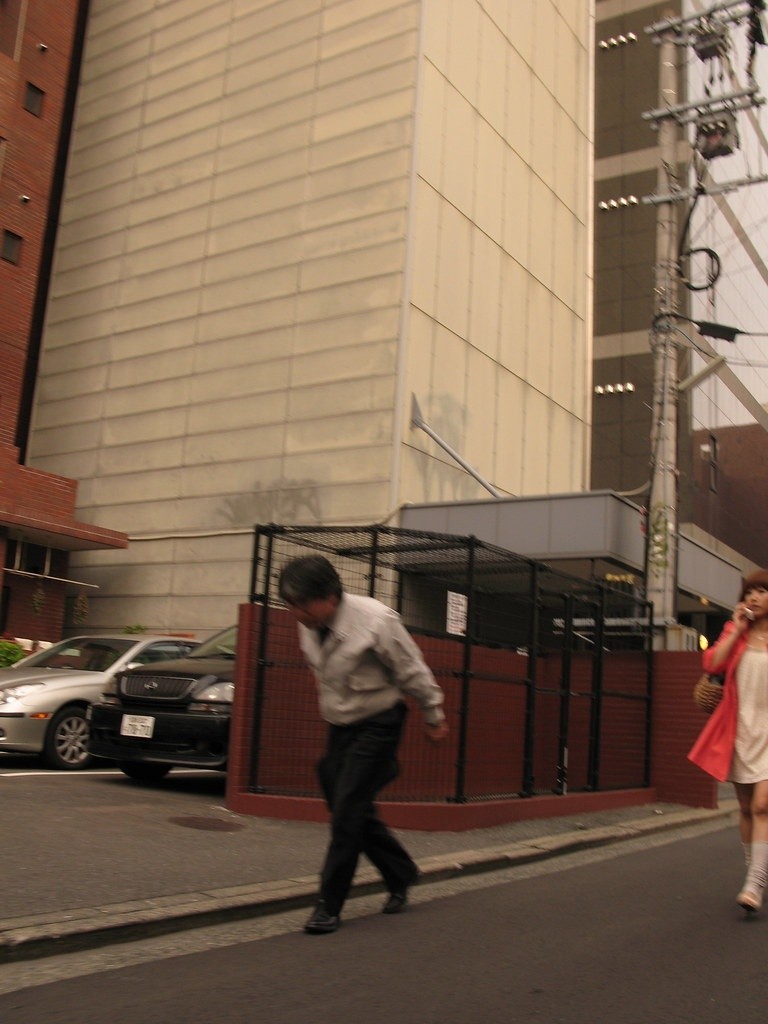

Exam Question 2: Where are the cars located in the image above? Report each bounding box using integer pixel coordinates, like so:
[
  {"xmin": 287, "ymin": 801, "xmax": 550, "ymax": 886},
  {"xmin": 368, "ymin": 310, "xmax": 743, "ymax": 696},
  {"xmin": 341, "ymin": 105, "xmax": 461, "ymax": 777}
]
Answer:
[{"xmin": 0, "ymin": 634, "xmax": 236, "ymax": 770}]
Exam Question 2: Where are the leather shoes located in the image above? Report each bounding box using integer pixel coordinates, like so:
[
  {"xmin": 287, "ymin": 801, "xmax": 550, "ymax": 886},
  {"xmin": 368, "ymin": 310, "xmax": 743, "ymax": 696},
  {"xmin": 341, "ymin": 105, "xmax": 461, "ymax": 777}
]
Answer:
[
  {"xmin": 384, "ymin": 865, "xmax": 417, "ymax": 913},
  {"xmin": 305, "ymin": 910, "xmax": 338, "ymax": 931}
]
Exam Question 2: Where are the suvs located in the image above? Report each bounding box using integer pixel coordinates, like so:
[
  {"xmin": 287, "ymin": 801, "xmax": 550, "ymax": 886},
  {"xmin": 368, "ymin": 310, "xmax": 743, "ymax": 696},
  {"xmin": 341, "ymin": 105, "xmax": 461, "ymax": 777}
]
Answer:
[{"xmin": 85, "ymin": 623, "xmax": 239, "ymax": 788}]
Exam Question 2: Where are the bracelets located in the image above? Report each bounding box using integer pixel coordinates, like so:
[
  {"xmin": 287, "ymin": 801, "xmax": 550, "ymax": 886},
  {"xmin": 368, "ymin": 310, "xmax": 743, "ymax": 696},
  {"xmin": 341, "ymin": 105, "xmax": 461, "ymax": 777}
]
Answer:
[{"xmin": 426, "ymin": 720, "xmax": 444, "ymax": 728}]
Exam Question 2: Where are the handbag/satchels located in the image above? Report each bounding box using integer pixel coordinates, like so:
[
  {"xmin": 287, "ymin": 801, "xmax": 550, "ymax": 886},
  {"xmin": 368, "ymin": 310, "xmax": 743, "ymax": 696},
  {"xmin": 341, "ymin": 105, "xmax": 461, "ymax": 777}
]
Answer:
[{"xmin": 694, "ymin": 671, "xmax": 723, "ymax": 714}]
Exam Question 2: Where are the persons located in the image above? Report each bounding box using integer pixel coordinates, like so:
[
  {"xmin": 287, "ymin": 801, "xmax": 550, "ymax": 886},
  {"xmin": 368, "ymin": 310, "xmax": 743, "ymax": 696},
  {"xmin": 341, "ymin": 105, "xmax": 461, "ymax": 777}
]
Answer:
[
  {"xmin": 278, "ymin": 556, "xmax": 448, "ymax": 933},
  {"xmin": 687, "ymin": 569, "xmax": 768, "ymax": 911}
]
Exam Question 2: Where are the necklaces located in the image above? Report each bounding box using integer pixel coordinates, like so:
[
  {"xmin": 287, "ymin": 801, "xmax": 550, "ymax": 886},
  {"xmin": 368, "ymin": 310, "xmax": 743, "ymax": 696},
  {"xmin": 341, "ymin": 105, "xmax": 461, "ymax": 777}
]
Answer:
[{"xmin": 751, "ymin": 628, "xmax": 768, "ymax": 642}]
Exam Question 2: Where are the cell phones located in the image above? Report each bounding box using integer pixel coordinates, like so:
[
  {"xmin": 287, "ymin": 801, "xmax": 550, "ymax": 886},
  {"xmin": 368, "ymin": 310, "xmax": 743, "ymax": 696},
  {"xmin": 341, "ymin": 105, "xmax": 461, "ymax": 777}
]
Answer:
[{"xmin": 743, "ymin": 606, "xmax": 754, "ymax": 621}]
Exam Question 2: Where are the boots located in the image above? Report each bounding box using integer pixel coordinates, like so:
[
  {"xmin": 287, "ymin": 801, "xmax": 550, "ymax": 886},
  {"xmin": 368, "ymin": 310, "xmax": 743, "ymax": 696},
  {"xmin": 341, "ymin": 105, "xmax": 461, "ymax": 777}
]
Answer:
[
  {"xmin": 736, "ymin": 840, "xmax": 768, "ymax": 912},
  {"xmin": 740, "ymin": 841, "xmax": 750, "ymax": 872}
]
